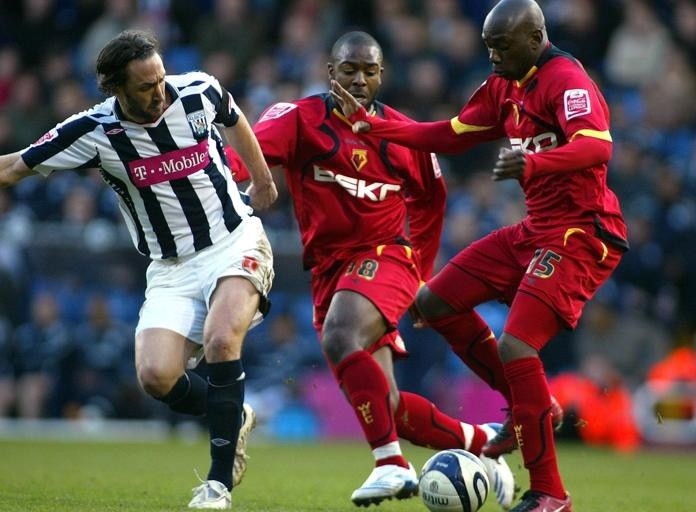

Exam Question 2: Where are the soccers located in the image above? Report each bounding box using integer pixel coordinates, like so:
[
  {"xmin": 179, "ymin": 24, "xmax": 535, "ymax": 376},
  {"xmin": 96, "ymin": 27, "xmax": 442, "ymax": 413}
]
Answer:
[{"xmin": 417, "ymin": 449, "xmax": 489, "ymax": 512}]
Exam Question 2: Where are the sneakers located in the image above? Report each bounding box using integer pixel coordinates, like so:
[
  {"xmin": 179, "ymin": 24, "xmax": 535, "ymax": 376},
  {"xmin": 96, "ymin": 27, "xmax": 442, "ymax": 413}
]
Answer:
[
  {"xmin": 231, "ymin": 401, "xmax": 257, "ymax": 488},
  {"xmin": 187, "ymin": 478, "xmax": 233, "ymax": 510},
  {"xmin": 350, "ymin": 461, "xmax": 420, "ymax": 508},
  {"xmin": 478, "ymin": 421, "xmax": 521, "ymax": 510},
  {"xmin": 505, "ymin": 488, "xmax": 573, "ymax": 512},
  {"xmin": 481, "ymin": 393, "xmax": 565, "ymax": 461}
]
